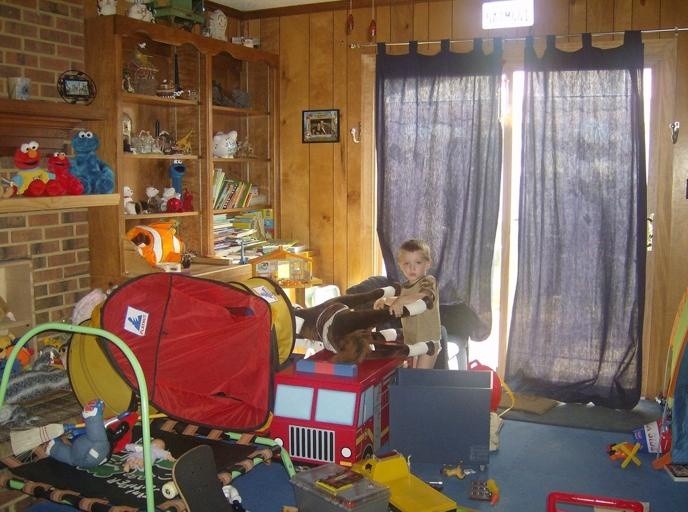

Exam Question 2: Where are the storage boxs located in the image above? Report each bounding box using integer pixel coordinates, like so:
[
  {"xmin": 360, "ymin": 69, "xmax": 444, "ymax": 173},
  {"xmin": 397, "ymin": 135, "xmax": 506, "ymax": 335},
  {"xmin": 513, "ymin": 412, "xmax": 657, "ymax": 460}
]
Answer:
[{"xmin": 288, "ymin": 462, "xmax": 390, "ymax": 512}]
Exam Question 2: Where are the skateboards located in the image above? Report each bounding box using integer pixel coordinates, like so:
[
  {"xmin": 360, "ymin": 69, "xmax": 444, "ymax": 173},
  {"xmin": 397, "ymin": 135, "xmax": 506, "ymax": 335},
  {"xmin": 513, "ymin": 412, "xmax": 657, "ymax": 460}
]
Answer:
[{"xmin": 162, "ymin": 442, "xmax": 237, "ymax": 511}]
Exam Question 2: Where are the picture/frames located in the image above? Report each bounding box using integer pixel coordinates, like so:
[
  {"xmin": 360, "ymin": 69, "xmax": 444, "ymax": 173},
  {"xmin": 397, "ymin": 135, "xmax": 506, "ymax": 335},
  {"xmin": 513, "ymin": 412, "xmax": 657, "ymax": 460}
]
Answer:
[{"xmin": 302, "ymin": 108, "xmax": 340, "ymax": 144}]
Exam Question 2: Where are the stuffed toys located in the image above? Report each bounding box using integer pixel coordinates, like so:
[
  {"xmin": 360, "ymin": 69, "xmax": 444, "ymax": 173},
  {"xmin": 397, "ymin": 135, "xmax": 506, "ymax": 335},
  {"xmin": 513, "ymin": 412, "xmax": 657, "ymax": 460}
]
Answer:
[
  {"xmin": 127, "ymin": 218, "xmax": 185, "ymax": 267},
  {"xmin": 290, "ymin": 281, "xmax": 437, "ymax": 364},
  {"xmin": 122, "ymin": 111, "xmax": 196, "ymax": 214},
  {"xmin": 123, "ymin": 438, "xmax": 166, "ymax": 474},
  {"xmin": 44, "ymin": 399, "xmax": 108, "ymax": 466},
  {"xmin": 3, "ymin": 127, "xmax": 115, "ymax": 197}
]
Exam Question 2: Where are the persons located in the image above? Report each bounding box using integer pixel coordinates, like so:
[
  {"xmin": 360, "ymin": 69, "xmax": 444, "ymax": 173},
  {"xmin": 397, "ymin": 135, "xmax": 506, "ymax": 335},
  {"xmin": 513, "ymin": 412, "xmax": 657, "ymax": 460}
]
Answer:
[
  {"xmin": 314, "ymin": 120, "xmax": 327, "ymax": 135},
  {"xmin": 374, "ymin": 239, "xmax": 442, "ymax": 370}
]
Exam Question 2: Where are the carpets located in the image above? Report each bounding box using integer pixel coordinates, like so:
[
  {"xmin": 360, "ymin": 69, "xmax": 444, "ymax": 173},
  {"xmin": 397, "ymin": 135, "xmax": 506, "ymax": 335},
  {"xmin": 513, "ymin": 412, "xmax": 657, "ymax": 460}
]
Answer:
[{"xmin": 498, "ymin": 401, "xmax": 662, "ymax": 434}]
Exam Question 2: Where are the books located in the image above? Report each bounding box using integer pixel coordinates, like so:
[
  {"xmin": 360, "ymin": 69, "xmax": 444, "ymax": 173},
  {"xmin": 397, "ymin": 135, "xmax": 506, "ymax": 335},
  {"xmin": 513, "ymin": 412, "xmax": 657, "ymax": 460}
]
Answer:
[
  {"xmin": 212, "ymin": 164, "xmax": 265, "ymax": 257},
  {"xmin": 663, "ymin": 464, "xmax": 688, "ymax": 482}
]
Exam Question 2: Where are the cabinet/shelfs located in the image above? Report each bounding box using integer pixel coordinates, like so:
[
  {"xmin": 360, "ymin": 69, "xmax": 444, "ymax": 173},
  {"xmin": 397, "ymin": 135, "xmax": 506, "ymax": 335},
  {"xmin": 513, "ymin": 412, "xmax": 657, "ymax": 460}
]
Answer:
[
  {"xmin": 84, "ymin": 15, "xmax": 280, "ymax": 290},
  {"xmin": 1, "ymin": 96, "xmax": 121, "ymax": 217}
]
